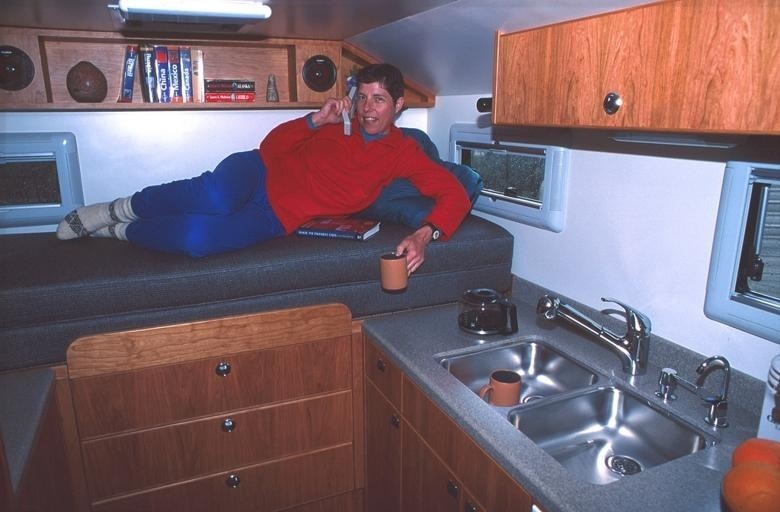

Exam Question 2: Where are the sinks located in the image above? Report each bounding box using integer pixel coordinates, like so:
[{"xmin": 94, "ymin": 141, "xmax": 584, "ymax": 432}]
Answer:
[
  {"xmin": 506, "ymin": 379, "xmax": 723, "ymax": 490},
  {"xmin": 430, "ymin": 333, "xmax": 605, "ymax": 415}
]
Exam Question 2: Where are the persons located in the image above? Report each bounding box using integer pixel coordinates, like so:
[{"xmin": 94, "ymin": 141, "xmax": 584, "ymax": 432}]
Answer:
[{"xmin": 52, "ymin": 62, "xmax": 476, "ymax": 279}]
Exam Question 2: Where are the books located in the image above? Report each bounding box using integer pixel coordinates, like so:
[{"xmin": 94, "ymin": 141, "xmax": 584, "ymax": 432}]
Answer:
[
  {"xmin": 118, "ymin": 41, "xmax": 259, "ymax": 108},
  {"xmin": 294, "ymin": 216, "xmax": 382, "ymax": 241}
]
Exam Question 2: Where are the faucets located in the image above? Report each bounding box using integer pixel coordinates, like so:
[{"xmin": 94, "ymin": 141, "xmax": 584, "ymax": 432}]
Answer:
[
  {"xmin": 694, "ymin": 355, "xmax": 731, "ymax": 427},
  {"xmin": 536, "ymin": 292, "xmax": 653, "ymax": 379}
]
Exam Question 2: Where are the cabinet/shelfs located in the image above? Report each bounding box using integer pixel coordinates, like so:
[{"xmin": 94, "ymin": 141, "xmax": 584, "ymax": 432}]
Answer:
[
  {"xmin": 362, "ymin": 334, "xmax": 548, "ymax": 512},
  {"xmin": 65, "ymin": 336, "xmax": 367, "ymax": 511},
  {"xmin": 0, "ymin": 26, "xmax": 435, "ymax": 111},
  {"xmin": 0, "ymin": 334, "xmax": 93, "ymax": 512},
  {"xmin": 492, "ymin": 0, "xmax": 780, "ymax": 136}
]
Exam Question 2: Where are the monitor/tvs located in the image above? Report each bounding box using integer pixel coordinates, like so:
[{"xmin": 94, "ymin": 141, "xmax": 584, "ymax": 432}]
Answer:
[{"xmin": 0, "ymin": 133, "xmax": 85, "ymax": 227}]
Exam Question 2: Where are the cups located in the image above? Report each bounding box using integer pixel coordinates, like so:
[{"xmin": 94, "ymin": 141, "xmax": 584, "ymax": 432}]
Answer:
[
  {"xmin": 380, "ymin": 252, "xmax": 410, "ymax": 294},
  {"xmin": 481, "ymin": 370, "xmax": 521, "ymax": 407}
]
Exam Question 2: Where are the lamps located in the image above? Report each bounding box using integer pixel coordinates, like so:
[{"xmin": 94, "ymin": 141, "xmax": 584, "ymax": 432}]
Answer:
[{"xmin": 108, "ymin": 0, "xmax": 273, "ymax": 23}]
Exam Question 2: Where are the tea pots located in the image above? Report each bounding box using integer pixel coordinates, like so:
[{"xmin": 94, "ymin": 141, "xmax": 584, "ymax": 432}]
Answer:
[{"xmin": 458, "ymin": 288, "xmax": 519, "ymax": 336}]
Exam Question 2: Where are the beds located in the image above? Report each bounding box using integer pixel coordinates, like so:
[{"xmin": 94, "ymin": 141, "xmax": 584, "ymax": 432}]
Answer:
[{"xmin": 0, "ymin": 210, "xmax": 515, "ymax": 372}]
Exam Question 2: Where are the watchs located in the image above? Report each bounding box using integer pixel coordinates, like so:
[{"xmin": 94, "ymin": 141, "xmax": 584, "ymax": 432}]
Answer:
[{"xmin": 428, "ymin": 222, "xmax": 443, "ymax": 242}]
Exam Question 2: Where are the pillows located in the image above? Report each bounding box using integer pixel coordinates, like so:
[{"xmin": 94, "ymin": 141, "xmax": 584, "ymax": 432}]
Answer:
[
  {"xmin": 350, "ymin": 159, "xmax": 483, "ymax": 228},
  {"xmin": 383, "ymin": 128, "xmax": 439, "ymax": 200}
]
